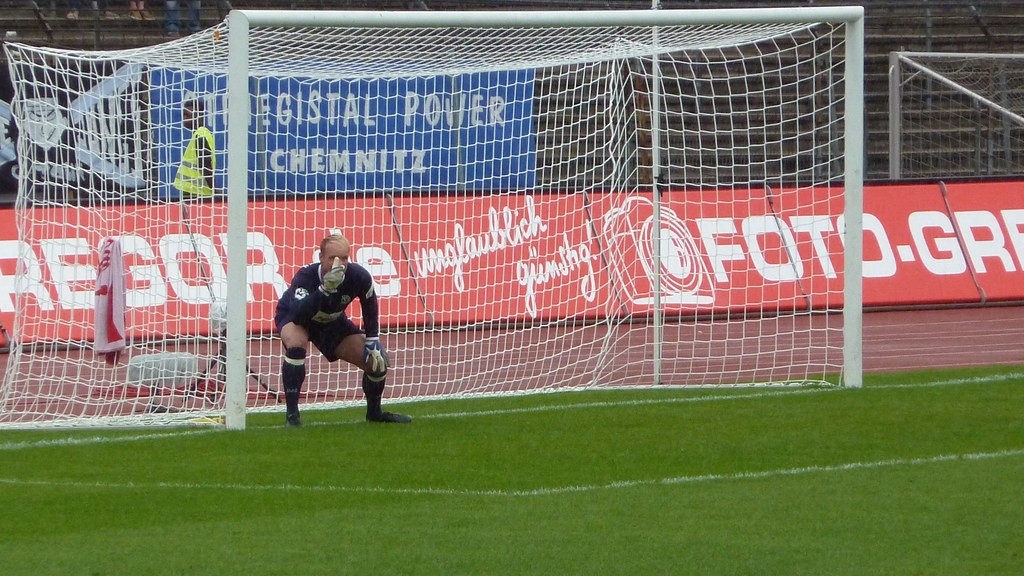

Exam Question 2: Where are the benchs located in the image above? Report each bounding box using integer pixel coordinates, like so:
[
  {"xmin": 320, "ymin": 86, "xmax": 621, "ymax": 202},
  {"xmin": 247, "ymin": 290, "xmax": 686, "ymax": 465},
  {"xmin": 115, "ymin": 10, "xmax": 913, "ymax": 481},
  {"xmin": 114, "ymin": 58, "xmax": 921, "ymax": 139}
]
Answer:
[{"xmin": 0, "ymin": 0, "xmax": 1024, "ymax": 194}]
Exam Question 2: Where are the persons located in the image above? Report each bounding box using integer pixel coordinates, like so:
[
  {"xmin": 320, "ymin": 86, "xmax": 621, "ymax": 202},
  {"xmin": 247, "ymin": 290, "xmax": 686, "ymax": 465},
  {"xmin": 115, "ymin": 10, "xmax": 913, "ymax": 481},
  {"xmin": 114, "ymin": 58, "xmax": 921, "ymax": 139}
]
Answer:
[
  {"xmin": 128, "ymin": 0, "xmax": 156, "ymax": 21},
  {"xmin": 171, "ymin": 100, "xmax": 215, "ymax": 203},
  {"xmin": 164, "ymin": 0, "xmax": 202, "ymax": 38},
  {"xmin": 274, "ymin": 234, "xmax": 412, "ymax": 427},
  {"xmin": 66, "ymin": 0, "xmax": 119, "ymax": 20}
]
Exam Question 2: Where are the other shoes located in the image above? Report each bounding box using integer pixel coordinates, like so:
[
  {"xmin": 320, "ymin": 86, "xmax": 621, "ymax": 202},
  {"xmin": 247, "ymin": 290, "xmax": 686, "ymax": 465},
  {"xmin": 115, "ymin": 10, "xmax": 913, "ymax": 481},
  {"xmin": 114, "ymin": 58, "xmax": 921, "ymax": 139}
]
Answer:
[
  {"xmin": 366, "ymin": 411, "xmax": 411, "ymax": 423},
  {"xmin": 286, "ymin": 412, "xmax": 301, "ymax": 425}
]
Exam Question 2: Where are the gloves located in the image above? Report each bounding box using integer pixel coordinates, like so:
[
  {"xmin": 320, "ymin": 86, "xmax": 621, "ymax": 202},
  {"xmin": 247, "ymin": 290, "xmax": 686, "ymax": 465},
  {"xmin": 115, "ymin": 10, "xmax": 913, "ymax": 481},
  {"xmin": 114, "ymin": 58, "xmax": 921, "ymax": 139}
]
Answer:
[
  {"xmin": 320, "ymin": 256, "xmax": 345, "ymax": 293},
  {"xmin": 364, "ymin": 339, "xmax": 391, "ymax": 373}
]
